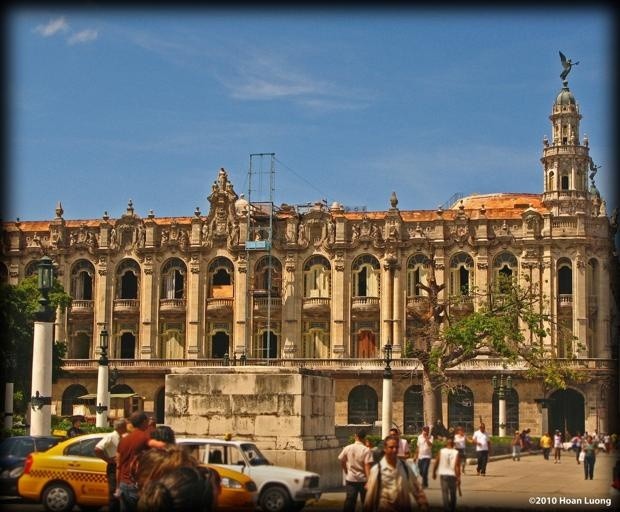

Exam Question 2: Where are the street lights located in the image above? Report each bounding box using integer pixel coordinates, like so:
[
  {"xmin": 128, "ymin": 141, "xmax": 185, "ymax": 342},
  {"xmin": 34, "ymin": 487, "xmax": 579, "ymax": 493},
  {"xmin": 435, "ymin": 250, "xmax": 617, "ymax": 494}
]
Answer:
[
  {"xmin": 26, "ymin": 253, "xmax": 61, "ymax": 438},
  {"xmin": 491, "ymin": 373, "xmax": 513, "ymax": 438},
  {"xmin": 94, "ymin": 322, "xmax": 112, "ymax": 427},
  {"xmin": 222, "ymin": 351, "xmax": 248, "ymax": 366},
  {"xmin": 380, "ymin": 336, "xmax": 394, "ymax": 440}
]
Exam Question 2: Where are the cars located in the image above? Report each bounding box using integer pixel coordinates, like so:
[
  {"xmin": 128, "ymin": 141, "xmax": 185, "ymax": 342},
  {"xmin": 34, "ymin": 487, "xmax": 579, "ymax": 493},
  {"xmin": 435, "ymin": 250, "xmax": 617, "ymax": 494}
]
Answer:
[
  {"xmin": 16, "ymin": 429, "xmax": 260, "ymax": 510},
  {"xmin": 0, "ymin": 434, "xmax": 65, "ymax": 496},
  {"xmin": 168, "ymin": 431, "xmax": 324, "ymax": 509}
]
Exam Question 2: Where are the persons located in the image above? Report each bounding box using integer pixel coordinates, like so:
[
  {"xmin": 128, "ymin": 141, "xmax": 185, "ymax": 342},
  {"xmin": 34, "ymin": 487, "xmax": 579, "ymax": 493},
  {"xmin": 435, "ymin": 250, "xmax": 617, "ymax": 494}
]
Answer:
[
  {"xmin": 92, "ymin": 409, "xmax": 222, "ymax": 511},
  {"xmin": 336, "ymin": 420, "xmax": 620, "ymax": 510},
  {"xmin": 63, "ymin": 418, "xmax": 86, "ymax": 439}
]
